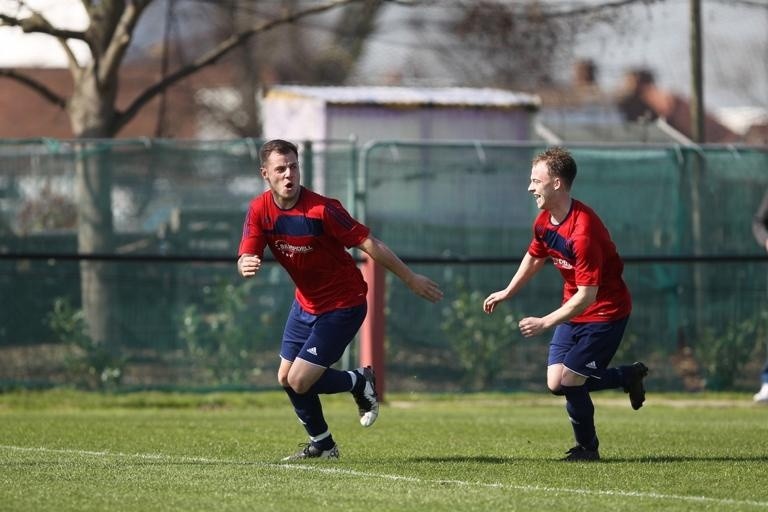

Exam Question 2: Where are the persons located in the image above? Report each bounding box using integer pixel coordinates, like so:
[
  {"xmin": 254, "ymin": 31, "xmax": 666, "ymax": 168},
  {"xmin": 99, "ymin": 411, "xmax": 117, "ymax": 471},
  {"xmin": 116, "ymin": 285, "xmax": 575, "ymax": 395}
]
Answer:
[
  {"xmin": 751, "ymin": 184, "xmax": 768, "ymax": 403},
  {"xmin": 235, "ymin": 138, "xmax": 444, "ymax": 466},
  {"xmin": 482, "ymin": 146, "xmax": 648, "ymax": 465}
]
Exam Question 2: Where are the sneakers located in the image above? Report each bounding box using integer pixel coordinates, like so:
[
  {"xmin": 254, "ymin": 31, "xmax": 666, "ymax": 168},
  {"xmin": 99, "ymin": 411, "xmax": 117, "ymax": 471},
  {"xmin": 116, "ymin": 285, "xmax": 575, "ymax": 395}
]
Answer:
[
  {"xmin": 353, "ymin": 365, "xmax": 379, "ymax": 428},
  {"xmin": 627, "ymin": 361, "xmax": 649, "ymax": 410},
  {"xmin": 281, "ymin": 440, "xmax": 341, "ymax": 462},
  {"xmin": 559, "ymin": 445, "xmax": 600, "ymax": 462}
]
